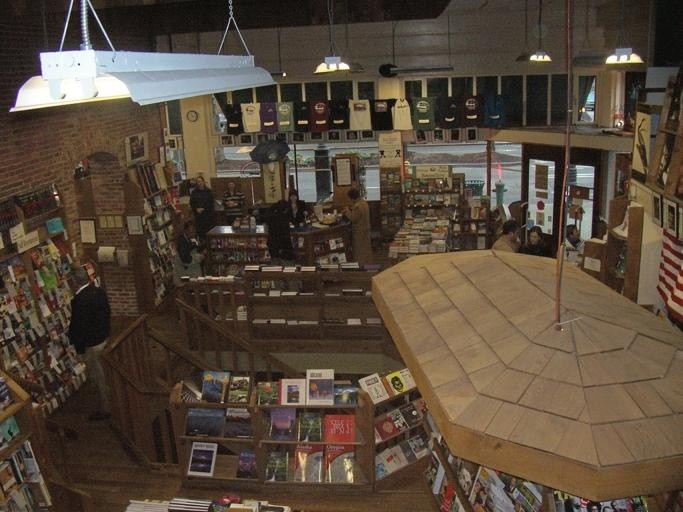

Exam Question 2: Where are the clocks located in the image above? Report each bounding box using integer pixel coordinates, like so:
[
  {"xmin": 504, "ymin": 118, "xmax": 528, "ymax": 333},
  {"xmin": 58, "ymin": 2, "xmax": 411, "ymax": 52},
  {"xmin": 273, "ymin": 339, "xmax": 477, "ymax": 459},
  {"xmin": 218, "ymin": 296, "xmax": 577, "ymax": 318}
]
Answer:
[{"xmin": 186, "ymin": 110, "xmax": 198, "ymax": 122}]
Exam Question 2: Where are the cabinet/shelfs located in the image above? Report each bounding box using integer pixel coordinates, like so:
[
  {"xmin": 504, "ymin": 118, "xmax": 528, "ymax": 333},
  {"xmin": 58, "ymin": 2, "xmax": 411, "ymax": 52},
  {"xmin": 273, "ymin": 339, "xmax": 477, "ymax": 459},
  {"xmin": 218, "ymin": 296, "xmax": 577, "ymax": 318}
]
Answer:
[
  {"xmin": 170, "ymin": 377, "xmax": 373, "ymax": 495},
  {"xmin": 207, "ymin": 218, "xmax": 355, "ymax": 265},
  {"xmin": 380, "ymin": 153, "xmax": 491, "ymax": 259},
  {"xmin": 187, "ymin": 265, "xmax": 387, "ymax": 341},
  {"xmin": 125, "ymin": 498, "xmax": 304, "ymax": 512},
  {"xmin": 331, "ymin": 155, "xmax": 367, "ymax": 202},
  {"xmin": 1, "ymin": 186, "xmax": 106, "ymax": 416},
  {"xmin": 435, "ymin": 455, "xmax": 547, "ymax": 512},
  {"xmin": 546, "ymin": 489, "xmax": 655, "ymax": 512},
  {"xmin": 361, "ymin": 365, "xmax": 444, "ymax": 493},
  {"xmin": 125, "ymin": 155, "xmax": 206, "ymax": 312},
  {"xmin": 1, "ymin": 374, "xmax": 61, "ymax": 510},
  {"xmin": 581, "ymin": 172, "xmax": 655, "ymax": 307}
]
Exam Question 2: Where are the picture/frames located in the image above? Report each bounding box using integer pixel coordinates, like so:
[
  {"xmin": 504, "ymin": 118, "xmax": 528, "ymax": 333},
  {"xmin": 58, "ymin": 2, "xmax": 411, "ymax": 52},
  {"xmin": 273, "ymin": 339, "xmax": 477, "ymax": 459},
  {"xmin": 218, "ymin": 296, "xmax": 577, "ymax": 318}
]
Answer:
[
  {"xmin": 220, "ymin": 124, "xmax": 479, "ymax": 147},
  {"xmin": 123, "ymin": 131, "xmax": 150, "ymax": 168},
  {"xmin": 659, "ymin": 192, "xmax": 679, "ymax": 239}
]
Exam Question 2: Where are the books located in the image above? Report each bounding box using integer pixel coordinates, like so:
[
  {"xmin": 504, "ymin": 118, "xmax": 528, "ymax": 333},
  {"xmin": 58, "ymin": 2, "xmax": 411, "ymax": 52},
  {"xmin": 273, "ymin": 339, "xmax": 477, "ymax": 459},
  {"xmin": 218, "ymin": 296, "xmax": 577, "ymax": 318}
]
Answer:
[
  {"xmin": 380, "ymin": 163, "xmax": 490, "ymax": 257},
  {"xmin": 214, "ymin": 236, "xmax": 347, "ymax": 279},
  {"xmin": 182, "ymin": 366, "xmax": 434, "ymax": 479},
  {"xmin": 429, "ymin": 435, "xmax": 647, "ymax": 510},
  {"xmin": 125, "ymin": 492, "xmax": 292, "ymax": 512},
  {"xmin": 179, "ymin": 257, "xmax": 393, "ymax": 331},
  {"xmin": 0, "ymin": 213, "xmax": 84, "ymax": 512},
  {"xmin": 129, "ymin": 161, "xmax": 184, "ymax": 305}
]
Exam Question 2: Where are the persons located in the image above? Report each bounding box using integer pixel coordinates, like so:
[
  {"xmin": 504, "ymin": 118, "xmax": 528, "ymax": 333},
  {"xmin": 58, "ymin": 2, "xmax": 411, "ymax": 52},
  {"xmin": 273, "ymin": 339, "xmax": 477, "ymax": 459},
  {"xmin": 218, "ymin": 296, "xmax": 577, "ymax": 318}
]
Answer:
[
  {"xmin": 174, "ymin": 220, "xmax": 202, "ymax": 296},
  {"xmin": 65, "ymin": 261, "xmax": 116, "ymax": 419},
  {"xmin": 556, "ymin": 224, "xmax": 584, "ymax": 266},
  {"xmin": 256, "ymin": 190, "xmax": 305, "ymax": 273},
  {"xmin": 341, "ymin": 187, "xmax": 375, "ymax": 264},
  {"xmin": 491, "ymin": 220, "xmax": 520, "ymax": 254},
  {"xmin": 222, "ymin": 182, "xmax": 244, "ymax": 224},
  {"xmin": 521, "ymin": 227, "xmax": 553, "ymax": 256},
  {"xmin": 189, "ymin": 175, "xmax": 213, "ymax": 232}
]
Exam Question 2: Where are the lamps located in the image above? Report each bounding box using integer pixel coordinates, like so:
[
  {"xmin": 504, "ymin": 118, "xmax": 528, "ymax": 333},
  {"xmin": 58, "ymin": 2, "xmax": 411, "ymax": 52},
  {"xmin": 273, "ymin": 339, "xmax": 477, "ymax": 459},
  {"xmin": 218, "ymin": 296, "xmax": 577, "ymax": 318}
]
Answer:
[
  {"xmin": 313, "ymin": 2, "xmax": 364, "ymax": 73},
  {"xmin": 8, "ymin": 2, "xmax": 278, "ymax": 112},
  {"xmin": 575, "ymin": 1, "xmax": 644, "ymax": 65},
  {"xmin": 515, "ymin": 1, "xmax": 550, "ymax": 62},
  {"xmin": 389, "ymin": 6, "xmax": 453, "ymax": 73},
  {"xmin": 268, "ymin": 30, "xmax": 287, "ymax": 78}
]
